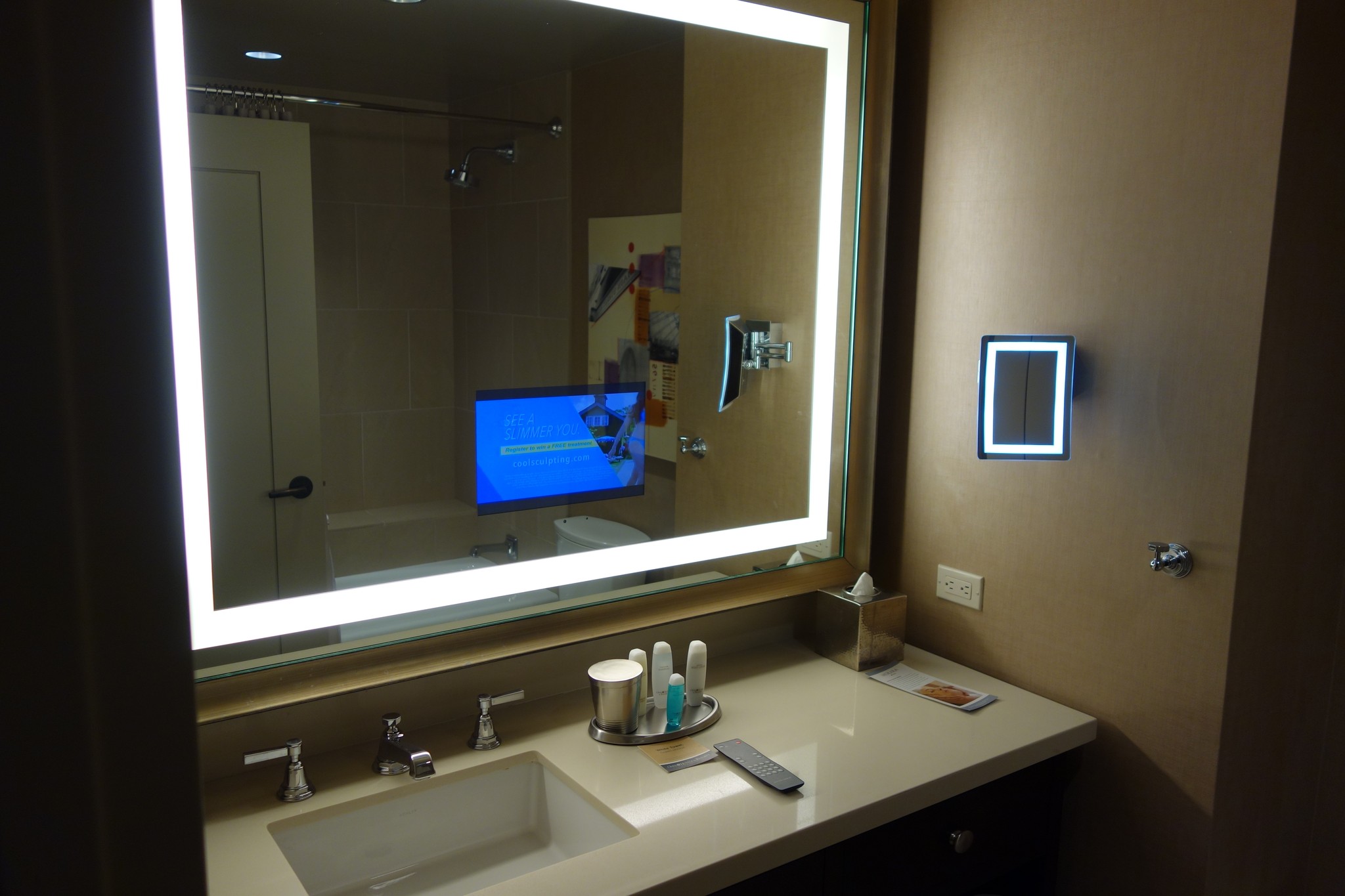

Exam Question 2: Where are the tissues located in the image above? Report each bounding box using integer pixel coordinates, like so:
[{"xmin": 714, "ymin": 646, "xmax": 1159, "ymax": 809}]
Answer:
[{"xmin": 812, "ymin": 572, "xmax": 908, "ymax": 672}]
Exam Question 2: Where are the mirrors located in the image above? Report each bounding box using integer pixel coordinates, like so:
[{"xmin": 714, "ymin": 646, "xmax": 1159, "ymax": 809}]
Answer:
[{"xmin": 148, "ymin": 1, "xmax": 867, "ymax": 684}]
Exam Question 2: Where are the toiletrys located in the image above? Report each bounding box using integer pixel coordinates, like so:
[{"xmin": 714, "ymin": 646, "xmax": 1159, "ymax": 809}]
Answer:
[{"xmin": 629, "ymin": 640, "xmax": 708, "ymax": 727}]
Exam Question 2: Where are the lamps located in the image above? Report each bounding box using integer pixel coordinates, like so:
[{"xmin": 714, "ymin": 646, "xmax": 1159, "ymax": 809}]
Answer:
[
  {"xmin": 977, "ymin": 334, "xmax": 1074, "ymax": 461},
  {"xmin": 717, "ymin": 314, "xmax": 794, "ymax": 412}
]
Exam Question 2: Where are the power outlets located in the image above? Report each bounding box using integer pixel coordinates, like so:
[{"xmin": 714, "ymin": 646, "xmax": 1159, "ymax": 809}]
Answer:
[{"xmin": 936, "ymin": 564, "xmax": 984, "ymax": 614}]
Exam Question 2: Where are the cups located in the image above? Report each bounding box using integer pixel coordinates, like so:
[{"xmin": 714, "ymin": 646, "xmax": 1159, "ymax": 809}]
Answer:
[{"xmin": 587, "ymin": 658, "xmax": 643, "ymax": 734}]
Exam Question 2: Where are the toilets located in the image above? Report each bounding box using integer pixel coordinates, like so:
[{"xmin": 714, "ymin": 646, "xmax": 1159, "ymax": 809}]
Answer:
[{"xmin": 553, "ymin": 515, "xmax": 652, "ymax": 556}]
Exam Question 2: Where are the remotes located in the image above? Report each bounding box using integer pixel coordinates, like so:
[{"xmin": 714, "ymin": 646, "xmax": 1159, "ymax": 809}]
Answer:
[{"xmin": 715, "ymin": 742, "xmax": 807, "ymax": 798}]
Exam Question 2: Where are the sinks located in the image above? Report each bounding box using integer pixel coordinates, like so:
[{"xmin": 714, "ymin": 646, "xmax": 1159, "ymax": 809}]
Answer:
[{"xmin": 267, "ymin": 748, "xmax": 639, "ymax": 896}]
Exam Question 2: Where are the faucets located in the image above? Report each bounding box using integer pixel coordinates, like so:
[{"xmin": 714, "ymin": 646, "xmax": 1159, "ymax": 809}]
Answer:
[
  {"xmin": 468, "ymin": 542, "xmax": 509, "ymax": 558},
  {"xmin": 371, "ymin": 712, "xmax": 438, "ymax": 781}
]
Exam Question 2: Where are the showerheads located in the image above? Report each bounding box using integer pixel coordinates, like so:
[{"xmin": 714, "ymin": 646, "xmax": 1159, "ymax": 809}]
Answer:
[{"xmin": 443, "ymin": 168, "xmax": 468, "ymax": 184}]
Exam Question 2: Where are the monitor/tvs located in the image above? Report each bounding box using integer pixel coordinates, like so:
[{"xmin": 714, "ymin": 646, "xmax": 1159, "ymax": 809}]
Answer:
[{"xmin": 468, "ymin": 380, "xmax": 651, "ymax": 516}]
